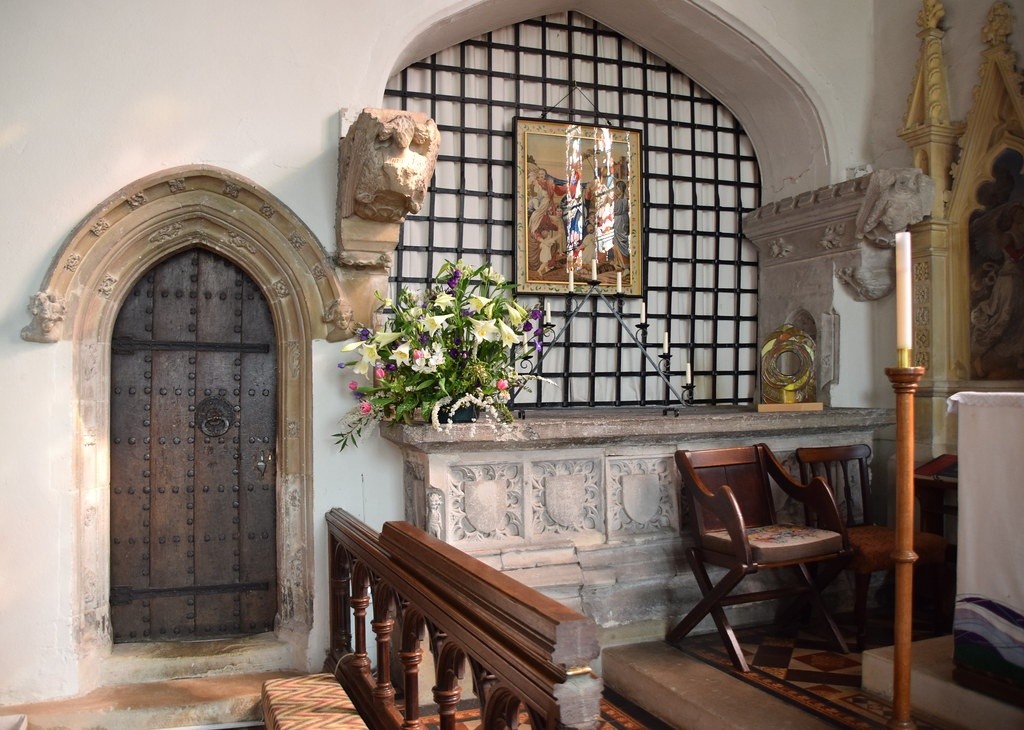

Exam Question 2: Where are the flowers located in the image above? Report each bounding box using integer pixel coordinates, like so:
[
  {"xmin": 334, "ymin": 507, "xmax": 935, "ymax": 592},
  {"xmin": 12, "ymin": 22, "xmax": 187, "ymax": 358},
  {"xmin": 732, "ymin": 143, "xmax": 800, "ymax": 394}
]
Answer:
[{"xmin": 329, "ymin": 257, "xmax": 561, "ymax": 454}]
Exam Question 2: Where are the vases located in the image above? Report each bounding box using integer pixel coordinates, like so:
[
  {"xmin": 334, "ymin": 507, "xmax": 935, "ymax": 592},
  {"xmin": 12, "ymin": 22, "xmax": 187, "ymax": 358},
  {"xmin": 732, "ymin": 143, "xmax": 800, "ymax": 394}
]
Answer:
[{"xmin": 428, "ymin": 378, "xmax": 482, "ymax": 424}]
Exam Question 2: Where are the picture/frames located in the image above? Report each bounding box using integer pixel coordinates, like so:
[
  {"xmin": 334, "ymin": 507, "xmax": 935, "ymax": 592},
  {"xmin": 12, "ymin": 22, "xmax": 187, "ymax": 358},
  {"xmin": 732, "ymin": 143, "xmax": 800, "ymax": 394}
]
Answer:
[{"xmin": 511, "ymin": 116, "xmax": 650, "ymax": 301}]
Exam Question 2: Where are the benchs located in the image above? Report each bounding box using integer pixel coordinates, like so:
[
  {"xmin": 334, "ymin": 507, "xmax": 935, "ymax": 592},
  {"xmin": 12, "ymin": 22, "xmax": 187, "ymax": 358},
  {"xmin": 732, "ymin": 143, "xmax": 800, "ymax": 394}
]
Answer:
[{"xmin": 257, "ymin": 668, "xmax": 369, "ymax": 730}]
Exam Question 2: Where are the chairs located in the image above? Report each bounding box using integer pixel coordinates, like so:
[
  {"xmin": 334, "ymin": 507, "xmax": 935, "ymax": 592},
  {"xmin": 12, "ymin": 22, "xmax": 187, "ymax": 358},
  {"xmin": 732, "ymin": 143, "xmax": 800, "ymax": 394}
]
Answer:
[
  {"xmin": 793, "ymin": 441, "xmax": 949, "ymax": 651},
  {"xmin": 664, "ymin": 441, "xmax": 850, "ymax": 676}
]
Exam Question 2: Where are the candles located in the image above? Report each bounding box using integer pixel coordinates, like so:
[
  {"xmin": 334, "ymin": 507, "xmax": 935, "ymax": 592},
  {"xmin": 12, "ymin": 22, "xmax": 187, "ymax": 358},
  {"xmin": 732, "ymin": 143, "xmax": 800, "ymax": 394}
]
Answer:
[
  {"xmin": 639, "ymin": 302, "xmax": 646, "ymax": 324},
  {"xmin": 568, "ymin": 270, "xmax": 575, "ymax": 291},
  {"xmin": 894, "ymin": 231, "xmax": 915, "ymax": 351},
  {"xmin": 544, "ymin": 302, "xmax": 551, "ymax": 323},
  {"xmin": 685, "ymin": 362, "xmax": 691, "ymax": 384},
  {"xmin": 521, "ymin": 332, "xmax": 528, "ymax": 355},
  {"xmin": 662, "ymin": 331, "xmax": 669, "ymax": 354},
  {"xmin": 590, "ymin": 258, "xmax": 598, "ymax": 281},
  {"xmin": 617, "ymin": 270, "xmax": 622, "ymax": 293}
]
